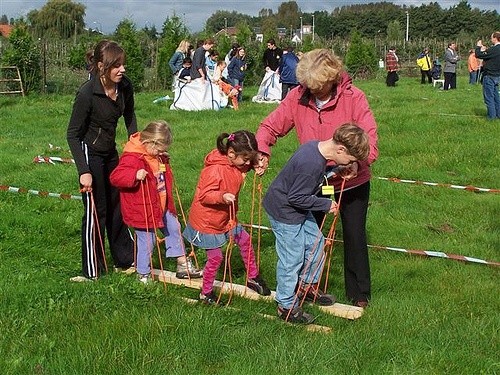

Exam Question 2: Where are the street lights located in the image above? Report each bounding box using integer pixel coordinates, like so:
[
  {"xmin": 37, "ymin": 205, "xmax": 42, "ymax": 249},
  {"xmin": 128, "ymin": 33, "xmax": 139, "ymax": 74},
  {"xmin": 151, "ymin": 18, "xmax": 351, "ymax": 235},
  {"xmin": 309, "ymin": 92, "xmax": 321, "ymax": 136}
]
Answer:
[
  {"xmin": 312, "ymin": 15, "xmax": 315, "ymax": 41},
  {"xmin": 405, "ymin": 11, "xmax": 409, "ymax": 41},
  {"xmin": 38, "ymin": 37, "xmax": 46, "ymax": 86},
  {"xmin": 300, "ymin": 17, "xmax": 302, "ymax": 45},
  {"xmin": 224, "ymin": 17, "xmax": 228, "ymax": 29},
  {"xmin": 93, "ymin": 21, "xmax": 102, "ymax": 31}
]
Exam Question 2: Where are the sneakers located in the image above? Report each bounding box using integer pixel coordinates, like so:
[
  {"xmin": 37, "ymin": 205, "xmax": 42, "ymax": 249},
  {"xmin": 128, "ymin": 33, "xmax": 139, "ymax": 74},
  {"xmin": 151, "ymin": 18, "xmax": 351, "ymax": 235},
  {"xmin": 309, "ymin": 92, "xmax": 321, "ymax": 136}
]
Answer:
[
  {"xmin": 277, "ymin": 304, "xmax": 313, "ymax": 324},
  {"xmin": 199, "ymin": 292, "xmax": 225, "ymax": 307},
  {"xmin": 247, "ymin": 278, "xmax": 271, "ymax": 296},
  {"xmin": 298, "ymin": 284, "xmax": 335, "ymax": 305}
]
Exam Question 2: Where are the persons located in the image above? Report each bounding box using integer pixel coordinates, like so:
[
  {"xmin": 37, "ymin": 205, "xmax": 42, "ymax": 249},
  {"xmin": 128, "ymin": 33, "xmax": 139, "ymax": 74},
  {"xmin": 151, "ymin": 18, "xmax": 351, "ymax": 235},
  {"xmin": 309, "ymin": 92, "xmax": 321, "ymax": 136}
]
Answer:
[
  {"xmin": 66, "ymin": 39, "xmax": 138, "ymax": 285},
  {"xmin": 295, "ymin": 52, "xmax": 305, "ymax": 61},
  {"xmin": 262, "ymin": 39, "xmax": 282, "ymax": 71},
  {"xmin": 417, "ymin": 48, "xmax": 434, "ymax": 84},
  {"xmin": 262, "ymin": 122, "xmax": 370, "ymax": 325},
  {"xmin": 212, "ymin": 62, "xmax": 239, "ymax": 111},
  {"xmin": 110, "ymin": 120, "xmax": 203, "ymax": 285},
  {"xmin": 386, "ymin": 46, "xmax": 400, "ymax": 86},
  {"xmin": 177, "ymin": 45, "xmax": 220, "ymax": 84},
  {"xmin": 169, "ymin": 39, "xmax": 191, "ymax": 77},
  {"xmin": 444, "ymin": 43, "xmax": 460, "ymax": 90},
  {"xmin": 221, "ymin": 43, "xmax": 240, "ymax": 83},
  {"xmin": 467, "ymin": 49, "xmax": 480, "ymax": 84},
  {"xmin": 476, "ymin": 46, "xmax": 486, "ymax": 84},
  {"xmin": 191, "ymin": 38, "xmax": 214, "ymax": 80},
  {"xmin": 182, "ymin": 131, "xmax": 271, "ymax": 307},
  {"xmin": 277, "ymin": 48, "xmax": 300, "ymax": 101},
  {"xmin": 475, "ymin": 31, "xmax": 500, "ymax": 119},
  {"xmin": 252, "ymin": 48, "xmax": 378, "ymax": 309},
  {"xmin": 227, "ymin": 48, "xmax": 249, "ymax": 102}
]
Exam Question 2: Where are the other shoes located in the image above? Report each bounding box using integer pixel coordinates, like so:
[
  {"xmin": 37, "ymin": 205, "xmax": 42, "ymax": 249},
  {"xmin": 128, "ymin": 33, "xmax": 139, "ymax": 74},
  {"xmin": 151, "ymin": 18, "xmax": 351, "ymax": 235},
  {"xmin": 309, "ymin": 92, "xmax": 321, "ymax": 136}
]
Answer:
[{"xmin": 355, "ymin": 300, "xmax": 368, "ymax": 308}]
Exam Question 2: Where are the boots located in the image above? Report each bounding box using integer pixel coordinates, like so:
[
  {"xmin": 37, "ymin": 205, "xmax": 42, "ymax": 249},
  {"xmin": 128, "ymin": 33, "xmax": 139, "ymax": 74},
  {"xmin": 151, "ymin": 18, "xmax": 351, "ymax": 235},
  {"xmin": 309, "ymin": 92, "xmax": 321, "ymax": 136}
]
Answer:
[
  {"xmin": 176, "ymin": 256, "xmax": 203, "ymax": 278},
  {"xmin": 137, "ymin": 274, "xmax": 151, "ymax": 284}
]
